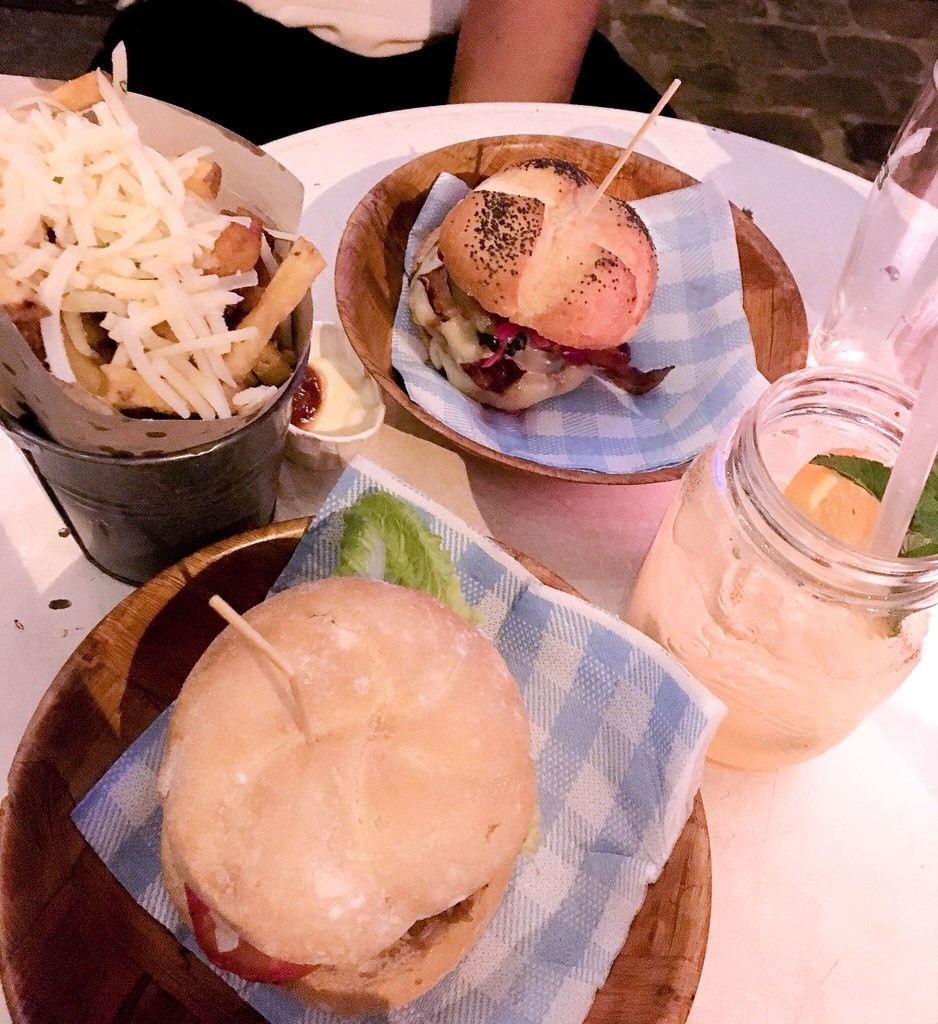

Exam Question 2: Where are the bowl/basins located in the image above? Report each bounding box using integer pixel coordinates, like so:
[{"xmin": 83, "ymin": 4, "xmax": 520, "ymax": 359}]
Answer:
[{"xmin": 286, "ymin": 320, "xmax": 384, "ymax": 473}]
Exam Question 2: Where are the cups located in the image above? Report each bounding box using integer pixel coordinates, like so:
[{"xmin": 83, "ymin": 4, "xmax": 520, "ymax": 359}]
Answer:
[
  {"xmin": 0, "ymin": 351, "xmax": 313, "ymax": 596},
  {"xmin": 624, "ymin": 367, "xmax": 938, "ymax": 771},
  {"xmin": 811, "ymin": 66, "xmax": 938, "ymax": 387}
]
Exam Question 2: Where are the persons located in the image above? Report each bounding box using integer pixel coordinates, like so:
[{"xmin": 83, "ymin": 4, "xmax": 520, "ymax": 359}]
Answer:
[{"xmin": 90, "ymin": 0, "xmax": 678, "ymax": 147}]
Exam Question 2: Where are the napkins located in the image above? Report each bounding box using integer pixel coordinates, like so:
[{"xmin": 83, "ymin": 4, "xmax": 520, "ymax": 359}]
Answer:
[
  {"xmin": 69, "ymin": 455, "xmax": 728, "ymax": 1024},
  {"xmin": 388, "ymin": 167, "xmax": 773, "ymax": 473}
]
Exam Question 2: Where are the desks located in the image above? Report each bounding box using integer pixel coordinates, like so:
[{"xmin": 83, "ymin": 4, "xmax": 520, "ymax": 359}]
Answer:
[{"xmin": 0, "ymin": 103, "xmax": 938, "ymax": 1024}]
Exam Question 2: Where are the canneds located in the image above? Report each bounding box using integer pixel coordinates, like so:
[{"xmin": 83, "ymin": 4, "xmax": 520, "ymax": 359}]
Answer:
[{"xmin": 623, "ymin": 368, "xmax": 938, "ymax": 771}]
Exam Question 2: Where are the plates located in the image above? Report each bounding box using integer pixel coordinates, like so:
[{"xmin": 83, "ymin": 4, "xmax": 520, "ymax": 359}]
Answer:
[
  {"xmin": 0, "ymin": 525, "xmax": 712, "ymax": 1024},
  {"xmin": 335, "ymin": 135, "xmax": 808, "ymax": 486}
]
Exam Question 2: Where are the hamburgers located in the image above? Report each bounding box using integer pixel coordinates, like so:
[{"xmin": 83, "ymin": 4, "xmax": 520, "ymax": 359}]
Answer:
[
  {"xmin": 155, "ymin": 488, "xmax": 537, "ymax": 1015},
  {"xmin": 407, "ymin": 157, "xmax": 676, "ymax": 411}
]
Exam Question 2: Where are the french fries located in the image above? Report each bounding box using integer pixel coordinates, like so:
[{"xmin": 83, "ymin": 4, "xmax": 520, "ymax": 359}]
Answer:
[{"xmin": 0, "ymin": 37, "xmax": 327, "ymax": 423}]
